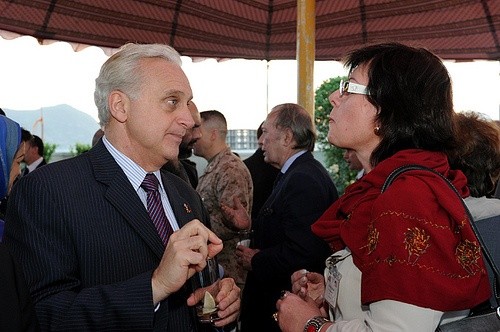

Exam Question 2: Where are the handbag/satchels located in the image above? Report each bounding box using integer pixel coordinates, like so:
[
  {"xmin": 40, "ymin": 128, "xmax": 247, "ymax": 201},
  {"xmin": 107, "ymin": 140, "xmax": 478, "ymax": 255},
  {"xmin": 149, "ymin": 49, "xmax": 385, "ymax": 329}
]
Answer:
[{"xmin": 380, "ymin": 164, "xmax": 500, "ymax": 332}]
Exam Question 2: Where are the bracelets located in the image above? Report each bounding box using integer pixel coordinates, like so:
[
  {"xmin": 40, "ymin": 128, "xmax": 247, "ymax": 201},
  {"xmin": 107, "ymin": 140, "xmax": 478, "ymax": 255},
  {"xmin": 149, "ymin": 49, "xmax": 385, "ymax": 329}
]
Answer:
[{"xmin": 303, "ymin": 316, "xmax": 328, "ymax": 332}]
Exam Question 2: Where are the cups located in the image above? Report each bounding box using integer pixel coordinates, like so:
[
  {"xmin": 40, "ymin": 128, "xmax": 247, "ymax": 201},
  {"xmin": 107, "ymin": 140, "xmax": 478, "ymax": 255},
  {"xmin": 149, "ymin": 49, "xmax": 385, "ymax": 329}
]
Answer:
[
  {"xmin": 238, "ymin": 229, "xmax": 255, "ymax": 249},
  {"xmin": 191, "ymin": 254, "xmax": 223, "ymax": 324}
]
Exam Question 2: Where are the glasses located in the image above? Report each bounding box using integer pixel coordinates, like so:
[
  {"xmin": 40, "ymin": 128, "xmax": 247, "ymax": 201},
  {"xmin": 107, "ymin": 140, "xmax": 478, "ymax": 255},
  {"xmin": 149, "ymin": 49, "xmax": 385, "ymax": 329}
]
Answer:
[{"xmin": 339, "ymin": 79, "xmax": 371, "ymax": 98}]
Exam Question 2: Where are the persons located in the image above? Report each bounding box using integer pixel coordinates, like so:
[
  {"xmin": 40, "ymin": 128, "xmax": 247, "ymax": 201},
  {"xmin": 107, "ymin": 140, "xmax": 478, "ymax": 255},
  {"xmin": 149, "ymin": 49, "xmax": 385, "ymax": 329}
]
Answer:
[
  {"xmin": 162, "ymin": 43, "xmax": 500, "ymax": 332},
  {"xmin": 6, "ymin": 42, "xmax": 240, "ymax": 332},
  {"xmin": 0, "ymin": 110, "xmax": 104, "ymax": 332}
]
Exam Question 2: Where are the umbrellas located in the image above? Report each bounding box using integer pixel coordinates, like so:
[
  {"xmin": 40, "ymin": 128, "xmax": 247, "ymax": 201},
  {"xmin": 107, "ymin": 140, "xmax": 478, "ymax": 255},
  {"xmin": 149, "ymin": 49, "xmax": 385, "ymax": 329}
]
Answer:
[{"xmin": 0, "ymin": 0, "xmax": 500, "ymax": 119}]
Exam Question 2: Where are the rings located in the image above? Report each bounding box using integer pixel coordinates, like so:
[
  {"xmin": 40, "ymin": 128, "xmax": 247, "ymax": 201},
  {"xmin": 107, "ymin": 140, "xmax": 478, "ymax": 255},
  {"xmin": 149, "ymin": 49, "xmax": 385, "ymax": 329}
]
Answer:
[{"xmin": 273, "ymin": 312, "xmax": 278, "ymax": 322}]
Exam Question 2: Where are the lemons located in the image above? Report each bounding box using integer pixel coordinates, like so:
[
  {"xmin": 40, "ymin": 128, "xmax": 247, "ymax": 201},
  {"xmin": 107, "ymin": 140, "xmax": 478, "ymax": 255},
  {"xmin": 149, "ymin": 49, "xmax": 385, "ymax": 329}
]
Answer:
[{"xmin": 203, "ymin": 290, "xmax": 216, "ymax": 314}]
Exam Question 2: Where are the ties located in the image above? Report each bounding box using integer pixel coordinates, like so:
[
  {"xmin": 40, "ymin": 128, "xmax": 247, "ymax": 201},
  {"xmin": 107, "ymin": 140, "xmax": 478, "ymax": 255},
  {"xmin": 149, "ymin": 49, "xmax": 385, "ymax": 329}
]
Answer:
[
  {"xmin": 141, "ymin": 173, "xmax": 175, "ymax": 248},
  {"xmin": 23, "ymin": 168, "xmax": 29, "ymax": 176}
]
